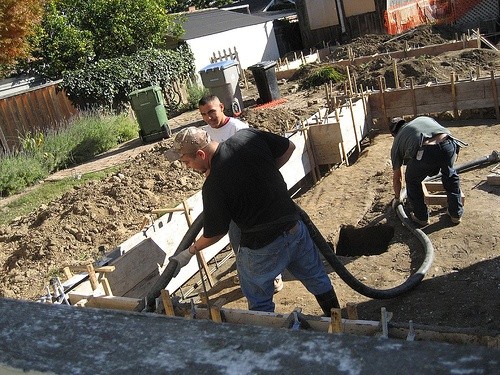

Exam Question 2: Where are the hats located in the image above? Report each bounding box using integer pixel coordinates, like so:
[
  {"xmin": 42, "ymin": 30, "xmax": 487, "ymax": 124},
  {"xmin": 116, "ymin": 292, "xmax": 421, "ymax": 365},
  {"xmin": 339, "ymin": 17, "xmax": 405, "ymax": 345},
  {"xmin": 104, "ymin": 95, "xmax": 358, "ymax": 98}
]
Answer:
[
  {"xmin": 388, "ymin": 117, "xmax": 406, "ymax": 131},
  {"xmin": 164, "ymin": 126, "xmax": 210, "ymax": 161}
]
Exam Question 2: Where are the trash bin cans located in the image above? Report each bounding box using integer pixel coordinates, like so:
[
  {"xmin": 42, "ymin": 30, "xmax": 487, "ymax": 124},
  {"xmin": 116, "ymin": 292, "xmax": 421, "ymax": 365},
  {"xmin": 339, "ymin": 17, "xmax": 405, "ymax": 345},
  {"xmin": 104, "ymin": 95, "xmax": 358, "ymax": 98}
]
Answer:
[
  {"xmin": 247, "ymin": 60, "xmax": 280, "ymax": 104},
  {"xmin": 127, "ymin": 87, "xmax": 172, "ymax": 145},
  {"xmin": 198, "ymin": 59, "xmax": 245, "ymax": 116}
]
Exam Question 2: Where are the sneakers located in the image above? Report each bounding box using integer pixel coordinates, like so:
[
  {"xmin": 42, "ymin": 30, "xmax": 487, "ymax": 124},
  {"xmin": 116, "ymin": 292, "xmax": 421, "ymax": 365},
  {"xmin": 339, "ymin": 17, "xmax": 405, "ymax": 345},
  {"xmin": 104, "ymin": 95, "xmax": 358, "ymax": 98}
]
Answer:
[
  {"xmin": 447, "ymin": 208, "xmax": 461, "ymax": 223},
  {"xmin": 411, "ymin": 213, "xmax": 430, "ymax": 226}
]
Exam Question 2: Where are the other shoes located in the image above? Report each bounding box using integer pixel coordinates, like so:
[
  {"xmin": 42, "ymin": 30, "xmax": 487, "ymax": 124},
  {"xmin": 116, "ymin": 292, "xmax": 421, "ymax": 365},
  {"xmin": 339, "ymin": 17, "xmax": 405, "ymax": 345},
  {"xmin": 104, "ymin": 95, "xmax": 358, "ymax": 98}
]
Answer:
[{"xmin": 273, "ymin": 273, "xmax": 283, "ymax": 293}]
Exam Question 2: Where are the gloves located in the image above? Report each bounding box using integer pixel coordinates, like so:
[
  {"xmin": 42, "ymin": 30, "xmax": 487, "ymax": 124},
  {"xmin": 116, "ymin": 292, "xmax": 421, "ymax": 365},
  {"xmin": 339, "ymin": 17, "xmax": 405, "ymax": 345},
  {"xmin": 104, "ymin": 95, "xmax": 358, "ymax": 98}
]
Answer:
[
  {"xmin": 392, "ymin": 198, "xmax": 400, "ymax": 214},
  {"xmin": 169, "ymin": 246, "xmax": 195, "ymax": 278}
]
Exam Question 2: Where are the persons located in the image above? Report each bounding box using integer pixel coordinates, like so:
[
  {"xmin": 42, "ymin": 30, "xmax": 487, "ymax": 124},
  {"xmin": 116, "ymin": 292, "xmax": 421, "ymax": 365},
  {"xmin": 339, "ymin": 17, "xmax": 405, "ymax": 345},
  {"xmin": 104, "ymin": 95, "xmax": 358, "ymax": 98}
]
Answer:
[
  {"xmin": 199, "ymin": 96, "xmax": 284, "ymax": 293},
  {"xmin": 168, "ymin": 127, "xmax": 340, "ymax": 318},
  {"xmin": 389, "ymin": 116, "xmax": 464, "ymax": 225}
]
